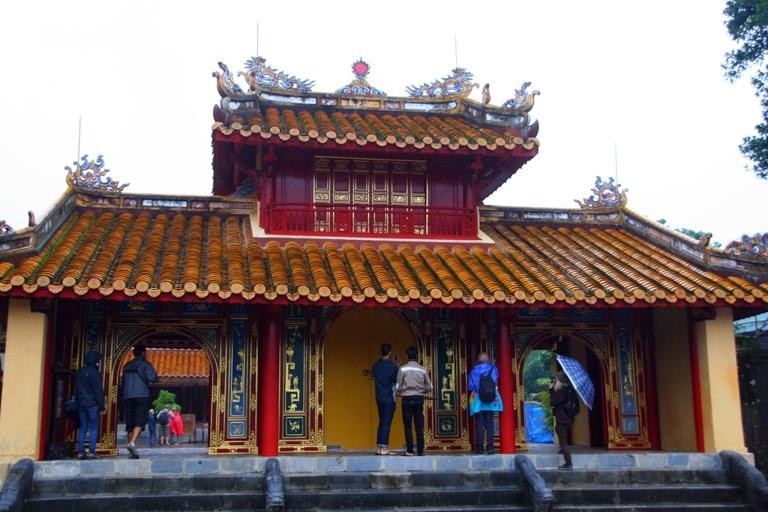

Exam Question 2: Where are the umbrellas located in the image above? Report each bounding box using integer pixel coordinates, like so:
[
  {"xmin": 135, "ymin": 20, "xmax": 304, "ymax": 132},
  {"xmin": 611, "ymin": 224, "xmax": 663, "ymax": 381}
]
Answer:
[{"xmin": 555, "ymin": 354, "xmax": 595, "ymax": 412}]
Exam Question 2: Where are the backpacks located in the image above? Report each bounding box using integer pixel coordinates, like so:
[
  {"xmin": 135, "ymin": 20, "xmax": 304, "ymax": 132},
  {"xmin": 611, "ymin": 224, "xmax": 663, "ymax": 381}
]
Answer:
[
  {"xmin": 159, "ymin": 414, "xmax": 169, "ymax": 425},
  {"xmin": 479, "ymin": 375, "xmax": 495, "ymax": 403},
  {"xmin": 565, "ymin": 385, "xmax": 580, "ymax": 417}
]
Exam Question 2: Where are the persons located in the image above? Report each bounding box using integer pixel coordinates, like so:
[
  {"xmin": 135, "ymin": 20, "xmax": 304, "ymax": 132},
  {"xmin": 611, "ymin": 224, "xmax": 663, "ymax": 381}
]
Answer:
[
  {"xmin": 371, "ymin": 343, "xmax": 401, "ymax": 456},
  {"xmin": 148, "ymin": 404, "xmax": 185, "ymax": 449},
  {"xmin": 395, "ymin": 346, "xmax": 433, "ymax": 457},
  {"xmin": 121, "ymin": 342, "xmax": 158, "ymax": 459},
  {"xmin": 467, "ymin": 352, "xmax": 503, "ymax": 454},
  {"xmin": 548, "ymin": 372, "xmax": 573, "ymax": 471},
  {"xmin": 75, "ymin": 351, "xmax": 106, "ymax": 459}
]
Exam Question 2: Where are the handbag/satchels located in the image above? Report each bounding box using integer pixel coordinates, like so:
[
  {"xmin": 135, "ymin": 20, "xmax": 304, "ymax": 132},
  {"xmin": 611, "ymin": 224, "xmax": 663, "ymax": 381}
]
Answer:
[{"xmin": 65, "ymin": 399, "xmax": 79, "ymax": 422}]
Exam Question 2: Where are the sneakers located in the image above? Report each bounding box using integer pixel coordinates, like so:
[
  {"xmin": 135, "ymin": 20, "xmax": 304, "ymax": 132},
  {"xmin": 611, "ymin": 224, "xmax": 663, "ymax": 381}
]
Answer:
[{"xmin": 126, "ymin": 442, "xmax": 139, "ymax": 459}]
